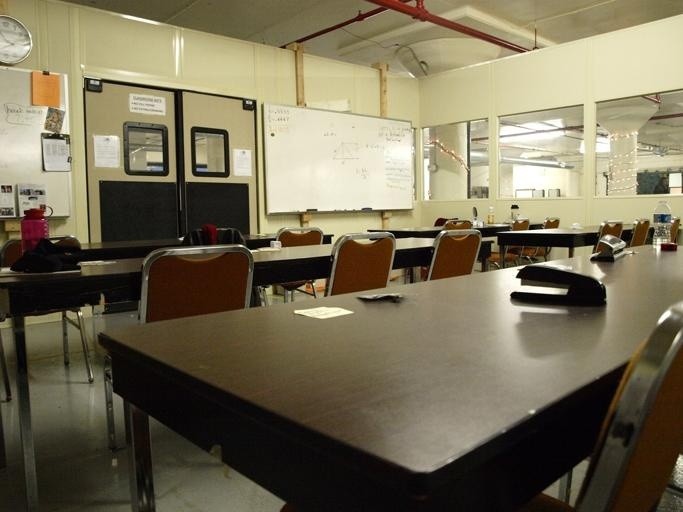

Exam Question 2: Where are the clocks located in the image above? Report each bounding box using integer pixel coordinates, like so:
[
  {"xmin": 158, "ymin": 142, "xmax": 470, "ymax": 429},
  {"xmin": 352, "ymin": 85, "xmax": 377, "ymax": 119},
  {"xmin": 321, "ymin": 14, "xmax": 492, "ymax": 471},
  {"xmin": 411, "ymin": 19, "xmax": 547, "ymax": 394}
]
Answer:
[{"xmin": 0, "ymin": 14, "xmax": 33, "ymax": 64}]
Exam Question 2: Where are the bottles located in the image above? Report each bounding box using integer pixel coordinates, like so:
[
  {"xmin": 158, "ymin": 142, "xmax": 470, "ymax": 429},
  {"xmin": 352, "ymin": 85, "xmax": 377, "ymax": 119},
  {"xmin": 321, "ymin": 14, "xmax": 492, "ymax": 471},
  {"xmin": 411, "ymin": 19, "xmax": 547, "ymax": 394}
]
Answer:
[
  {"xmin": 487, "ymin": 207, "xmax": 493, "ymax": 224},
  {"xmin": 20, "ymin": 205, "xmax": 54, "ymax": 254},
  {"xmin": 511, "ymin": 204, "xmax": 519, "ymax": 223},
  {"xmin": 651, "ymin": 201, "xmax": 672, "ymax": 248}
]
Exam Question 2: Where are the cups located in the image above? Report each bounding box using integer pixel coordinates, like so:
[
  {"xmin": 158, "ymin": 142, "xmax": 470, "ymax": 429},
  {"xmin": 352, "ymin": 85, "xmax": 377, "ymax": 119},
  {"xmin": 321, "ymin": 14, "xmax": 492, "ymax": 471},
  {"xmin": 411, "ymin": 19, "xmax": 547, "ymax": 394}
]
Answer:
[{"xmin": 471, "ymin": 217, "xmax": 484, "ymax": 228}]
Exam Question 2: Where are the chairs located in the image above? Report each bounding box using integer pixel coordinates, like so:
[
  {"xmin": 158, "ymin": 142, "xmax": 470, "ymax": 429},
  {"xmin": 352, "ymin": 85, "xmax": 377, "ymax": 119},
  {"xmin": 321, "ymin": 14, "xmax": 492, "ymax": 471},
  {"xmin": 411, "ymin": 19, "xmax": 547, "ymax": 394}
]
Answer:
[
  {"xmin": 324, "ymin": 232, "xmax": 396, "ymax": 297},
  {"xmin": 276, "ymin": 226, "xmax": 323, "ymax": 304},
  {"xmin": 424, "ymin": 219, "xmax": 681, "ymax": 280},
  {"xmin": 515, "ymin": 300, "xmax": 683, "ymax": 510}
]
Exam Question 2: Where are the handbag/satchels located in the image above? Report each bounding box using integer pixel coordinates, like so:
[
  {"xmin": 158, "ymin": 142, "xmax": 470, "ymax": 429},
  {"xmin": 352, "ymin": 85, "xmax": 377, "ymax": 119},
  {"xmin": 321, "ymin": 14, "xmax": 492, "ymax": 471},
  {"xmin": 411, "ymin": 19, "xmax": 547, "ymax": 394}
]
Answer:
[{"xmin": 10, "ymin": 236, "xmax": 82, "ymax": 272}]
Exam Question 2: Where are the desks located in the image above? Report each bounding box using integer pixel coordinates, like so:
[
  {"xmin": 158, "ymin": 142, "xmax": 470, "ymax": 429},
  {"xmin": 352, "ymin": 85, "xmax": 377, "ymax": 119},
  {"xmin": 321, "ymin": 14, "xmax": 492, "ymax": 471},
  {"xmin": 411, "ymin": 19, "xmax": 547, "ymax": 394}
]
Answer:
[
  {"xmin": 1, "ymin": 236, "xmax": 493, "ymax": 511},
  {"xmin": 98, "ymin": 243, "xmax": 682, "ymax": 511}
]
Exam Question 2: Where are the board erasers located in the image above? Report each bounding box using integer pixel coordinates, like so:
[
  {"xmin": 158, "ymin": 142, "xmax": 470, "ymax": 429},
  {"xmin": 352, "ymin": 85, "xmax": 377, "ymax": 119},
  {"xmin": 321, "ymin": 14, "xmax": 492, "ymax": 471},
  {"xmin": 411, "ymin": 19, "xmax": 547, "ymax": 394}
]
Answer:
[
  {"xmin": 362, "ymin": 208, "xmax": 372, "ymax": 211},
  {"xmin": 307, "ymin": 209, "xmax": 318, "ymax": 211}
]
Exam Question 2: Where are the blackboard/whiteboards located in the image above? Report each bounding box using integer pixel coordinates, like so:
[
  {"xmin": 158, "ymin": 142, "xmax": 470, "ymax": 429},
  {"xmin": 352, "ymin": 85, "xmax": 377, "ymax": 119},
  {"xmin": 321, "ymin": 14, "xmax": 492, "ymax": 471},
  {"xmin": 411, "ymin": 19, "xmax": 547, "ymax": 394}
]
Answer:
[
  {"xmin": 262, "ymin": 102, "xmax": 415, "ymax": 216},
  {"xmin": 0, "ymin": 65, "xmax": 72, "ymax": 219}
]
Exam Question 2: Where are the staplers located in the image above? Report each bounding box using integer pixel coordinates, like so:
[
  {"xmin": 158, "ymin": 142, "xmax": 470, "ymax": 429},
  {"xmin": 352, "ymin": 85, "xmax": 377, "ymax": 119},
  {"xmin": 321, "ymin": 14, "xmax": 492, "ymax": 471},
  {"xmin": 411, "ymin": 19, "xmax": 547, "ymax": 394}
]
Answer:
[{"xmin": 510, "ymin": 266, "xmax": 606, "ymax": 307}]
